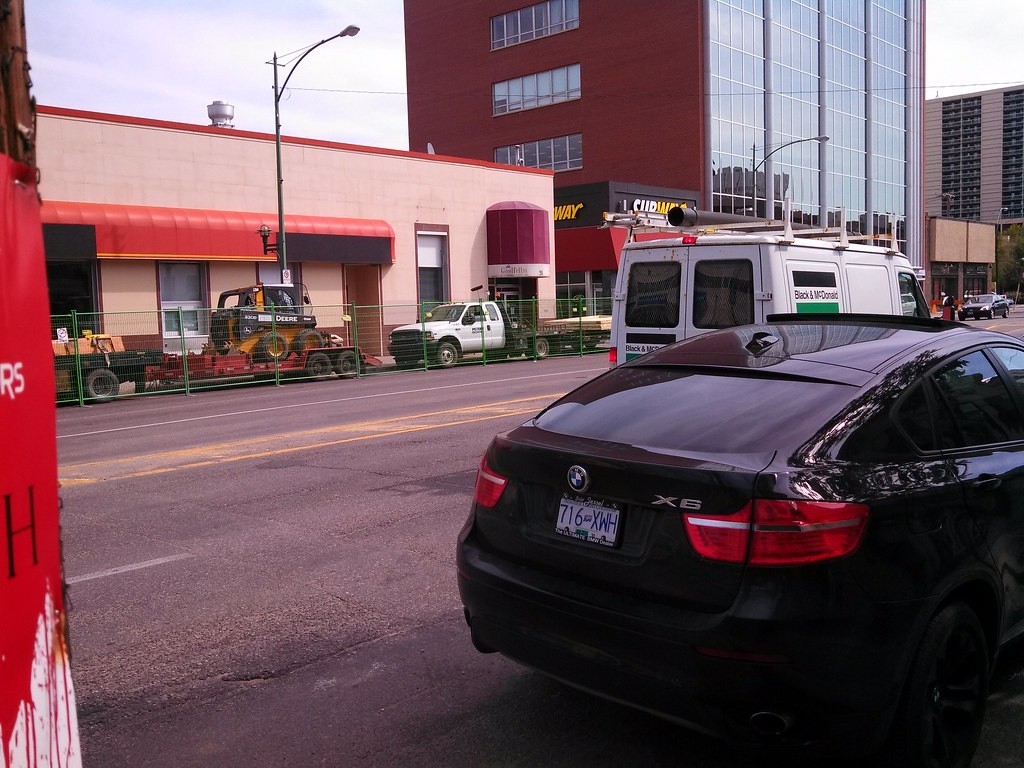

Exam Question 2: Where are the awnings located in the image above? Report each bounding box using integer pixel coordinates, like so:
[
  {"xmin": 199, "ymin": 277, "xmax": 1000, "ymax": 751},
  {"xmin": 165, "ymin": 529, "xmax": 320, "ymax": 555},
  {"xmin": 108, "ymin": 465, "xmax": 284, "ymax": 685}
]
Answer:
[{"xmin": 39, "ymin": 199, "xmax": 396, "ymax": 264}]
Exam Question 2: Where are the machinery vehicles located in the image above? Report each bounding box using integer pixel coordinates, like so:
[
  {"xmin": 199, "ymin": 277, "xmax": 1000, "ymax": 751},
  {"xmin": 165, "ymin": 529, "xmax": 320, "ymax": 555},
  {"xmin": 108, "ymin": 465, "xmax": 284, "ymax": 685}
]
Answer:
[{"xmin": 202, "ymin": 282, "xmax": 343, "ymax": 363}]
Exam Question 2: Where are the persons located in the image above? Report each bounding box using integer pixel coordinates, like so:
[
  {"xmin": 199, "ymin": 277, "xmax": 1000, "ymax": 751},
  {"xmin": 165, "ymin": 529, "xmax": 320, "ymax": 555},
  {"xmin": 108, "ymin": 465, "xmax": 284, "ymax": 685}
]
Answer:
[{"xmin": 943, "ymin": 292, "xmax": 954, "ymax": 308}]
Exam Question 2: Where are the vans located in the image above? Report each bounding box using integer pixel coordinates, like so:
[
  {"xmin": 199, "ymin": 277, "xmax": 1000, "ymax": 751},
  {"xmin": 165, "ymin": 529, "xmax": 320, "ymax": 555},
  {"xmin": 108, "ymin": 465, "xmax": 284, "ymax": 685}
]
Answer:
[{"xmin": 602, "ymin": 205, "xmax": 933, "ymax": 369}]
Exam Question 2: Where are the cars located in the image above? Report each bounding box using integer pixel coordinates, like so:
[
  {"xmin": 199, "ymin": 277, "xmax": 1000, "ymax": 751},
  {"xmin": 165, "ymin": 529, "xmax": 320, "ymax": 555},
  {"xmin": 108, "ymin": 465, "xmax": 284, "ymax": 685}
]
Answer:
[
  {"xmin": 959, "ymin": 294, "xmax": 1010, "ymax": 321},
  {"xmin": 454, "ymin": 313, "xmax": 1024, "ymax": 768}
]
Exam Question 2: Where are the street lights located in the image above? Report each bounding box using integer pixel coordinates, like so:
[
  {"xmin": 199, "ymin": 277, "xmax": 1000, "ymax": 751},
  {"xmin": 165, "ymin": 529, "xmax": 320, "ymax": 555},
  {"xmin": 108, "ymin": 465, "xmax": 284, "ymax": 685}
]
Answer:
[
  {"xmin": 271, "ymin": 27, "xmax": 364, "ymax": 284},
  {"xmin": 996, "ymin": 207, "xmax": 1008, "ymax": 234},
  {"xmin": 753, "ymin": 135, "xmax": 829, "ymax": 218}
]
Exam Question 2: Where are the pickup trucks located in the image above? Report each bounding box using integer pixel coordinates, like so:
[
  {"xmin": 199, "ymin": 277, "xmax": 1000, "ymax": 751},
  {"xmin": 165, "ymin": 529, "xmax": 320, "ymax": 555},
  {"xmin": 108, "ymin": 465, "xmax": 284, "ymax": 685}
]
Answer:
[{"xmin": 386, "ymin": 301, "xmax": 566, "ymax": 366}]
